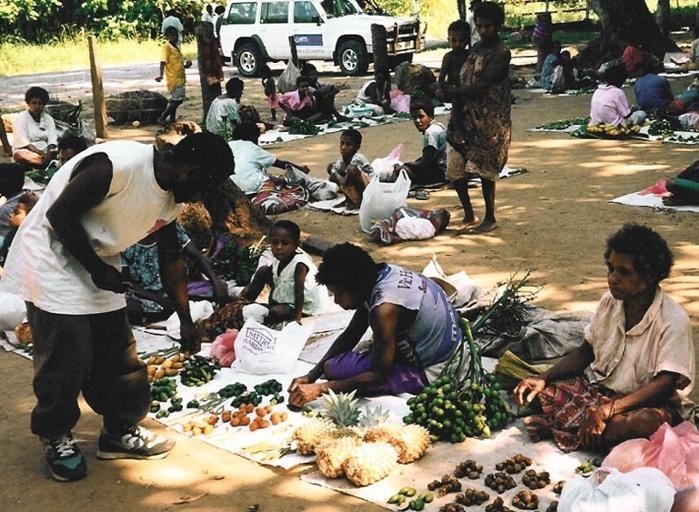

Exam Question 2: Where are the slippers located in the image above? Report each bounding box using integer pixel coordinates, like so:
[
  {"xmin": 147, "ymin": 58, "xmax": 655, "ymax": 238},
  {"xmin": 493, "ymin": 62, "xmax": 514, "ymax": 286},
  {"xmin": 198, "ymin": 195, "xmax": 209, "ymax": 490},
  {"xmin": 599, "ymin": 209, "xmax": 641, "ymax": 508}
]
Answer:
[
  {"xmin": 416, "ymin": 188, "xmax": 430, "ymax": 201},
  {"xmin": 408, "ymin": 191, "xmax": 416, "ymax": 198}
]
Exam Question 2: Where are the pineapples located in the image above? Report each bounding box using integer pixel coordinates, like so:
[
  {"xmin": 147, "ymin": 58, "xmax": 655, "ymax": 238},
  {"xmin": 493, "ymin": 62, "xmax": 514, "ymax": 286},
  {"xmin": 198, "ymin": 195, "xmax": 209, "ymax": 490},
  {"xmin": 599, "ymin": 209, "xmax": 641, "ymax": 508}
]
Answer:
[{"xmin": 292, "ymin": 388, "xmax": 431, "ymax": 487}]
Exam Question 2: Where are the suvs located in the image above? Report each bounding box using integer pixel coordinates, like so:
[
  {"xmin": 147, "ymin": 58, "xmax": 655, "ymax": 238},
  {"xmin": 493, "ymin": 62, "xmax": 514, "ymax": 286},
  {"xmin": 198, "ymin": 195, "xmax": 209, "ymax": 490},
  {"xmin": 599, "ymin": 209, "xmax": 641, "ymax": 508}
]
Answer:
[{"xmin": 217, "ymin": 1, "xmax": 419, "ymax": 77}]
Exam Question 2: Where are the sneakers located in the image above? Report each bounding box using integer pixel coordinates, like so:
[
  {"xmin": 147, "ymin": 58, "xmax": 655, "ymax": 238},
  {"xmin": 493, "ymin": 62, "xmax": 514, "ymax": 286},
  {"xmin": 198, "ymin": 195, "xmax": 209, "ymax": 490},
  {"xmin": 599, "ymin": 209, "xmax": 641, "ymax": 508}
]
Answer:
[
  {"xmin": 95, "ymin": 421, "xmax": 177, "ymax": 461},
  {"xmin": 37, "ymin": 432, "xmax": 90, "ymax": 482}
]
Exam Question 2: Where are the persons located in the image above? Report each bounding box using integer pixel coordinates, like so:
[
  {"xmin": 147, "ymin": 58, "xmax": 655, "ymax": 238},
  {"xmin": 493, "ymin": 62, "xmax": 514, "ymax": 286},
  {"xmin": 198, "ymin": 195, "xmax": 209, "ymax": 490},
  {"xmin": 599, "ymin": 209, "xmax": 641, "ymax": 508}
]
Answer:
[
  {"xmin": 1, "ymin": 0, "xmax": 509, "ymax": 326},
  {"xmin": 533, "ymin": 8, "xmax": 698, "ymax": 138},
  {"xmin": 0, "ymin": 132, "xmax": 234, "ymax": 483},
  {"xmin": 512, "ymin": 222, "xmax": 696, "ymax": 455},
  {"xmin": 287, "ymin": 240, "xmax": 463, "ymax": 409}
]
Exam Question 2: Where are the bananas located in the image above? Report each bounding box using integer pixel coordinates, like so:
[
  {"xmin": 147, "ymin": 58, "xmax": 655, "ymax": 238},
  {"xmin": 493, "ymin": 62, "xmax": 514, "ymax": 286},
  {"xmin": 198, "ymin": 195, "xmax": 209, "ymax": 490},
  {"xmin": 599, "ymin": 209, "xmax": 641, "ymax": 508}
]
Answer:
[{"xmin": 586, "ymin": 122, "xmax": 640, "ymax": 136}]
